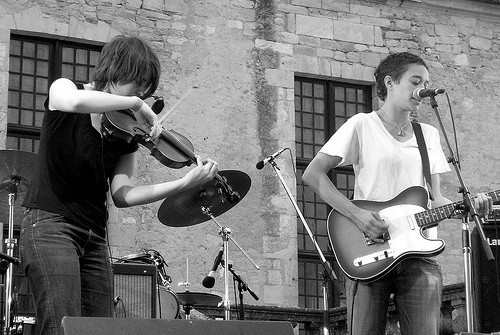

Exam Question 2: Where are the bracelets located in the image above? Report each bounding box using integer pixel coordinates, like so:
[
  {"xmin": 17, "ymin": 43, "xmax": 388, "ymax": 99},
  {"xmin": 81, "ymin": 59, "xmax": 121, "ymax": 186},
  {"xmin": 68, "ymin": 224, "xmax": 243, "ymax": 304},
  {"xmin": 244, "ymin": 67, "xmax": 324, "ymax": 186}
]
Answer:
[{"xmin": 130, "ymin": 98, "xmax": 143, "ymax": 112}]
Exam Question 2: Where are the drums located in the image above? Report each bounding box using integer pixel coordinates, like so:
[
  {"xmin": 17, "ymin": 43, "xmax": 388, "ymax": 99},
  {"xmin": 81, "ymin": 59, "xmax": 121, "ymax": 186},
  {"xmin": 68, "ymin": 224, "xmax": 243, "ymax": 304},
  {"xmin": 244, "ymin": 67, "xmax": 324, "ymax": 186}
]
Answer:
[{"xmin": 116, "ymin": 253, "xmax": 180, "ymax": 320}]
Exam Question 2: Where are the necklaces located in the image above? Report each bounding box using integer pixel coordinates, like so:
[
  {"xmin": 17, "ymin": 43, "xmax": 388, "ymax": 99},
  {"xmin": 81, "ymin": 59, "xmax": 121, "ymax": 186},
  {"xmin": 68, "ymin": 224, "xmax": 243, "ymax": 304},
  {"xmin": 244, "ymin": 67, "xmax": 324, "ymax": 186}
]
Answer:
[{"xmin": 380, "ymin": 108, "xmax": 409, "ymax": 137}]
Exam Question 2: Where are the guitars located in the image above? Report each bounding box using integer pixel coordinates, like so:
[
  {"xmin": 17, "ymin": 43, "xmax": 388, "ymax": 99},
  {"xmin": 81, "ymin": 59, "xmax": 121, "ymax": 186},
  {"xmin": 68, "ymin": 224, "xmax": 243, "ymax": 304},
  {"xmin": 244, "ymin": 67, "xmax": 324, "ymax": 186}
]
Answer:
[{"xmin": 326, "ymin": 185, "xmax": 500, "ymax": 284}]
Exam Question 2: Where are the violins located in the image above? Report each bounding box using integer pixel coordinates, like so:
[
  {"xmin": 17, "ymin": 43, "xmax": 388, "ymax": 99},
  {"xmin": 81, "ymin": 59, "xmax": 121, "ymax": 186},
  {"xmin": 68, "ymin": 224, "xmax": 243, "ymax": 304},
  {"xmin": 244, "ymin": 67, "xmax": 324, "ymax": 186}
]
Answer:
[{"xmin": 100, "ymin": 109, "xmax": 241, "ymax": 205}]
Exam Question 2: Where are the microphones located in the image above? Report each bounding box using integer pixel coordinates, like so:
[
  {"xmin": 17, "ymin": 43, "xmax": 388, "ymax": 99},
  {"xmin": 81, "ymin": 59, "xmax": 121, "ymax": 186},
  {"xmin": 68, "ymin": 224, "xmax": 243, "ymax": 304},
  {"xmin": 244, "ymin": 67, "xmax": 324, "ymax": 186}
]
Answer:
[
  {"xmin": 256, "ymin": 148, "xmax": 286, "ymax": 170},
  {"xmin": 202, "ymin": 245, "xmax": 224, "ymax": 288},
  {"xmin": 413, "ymin": 88, "xmax": 445, "ymax": 101},
  {"xmin": 0, "ymin": 252, "xmax": 21, "ymax": 265},
  {"xmin": 114, "ymin": 296, "xmax": 120, "ymax": 308}
]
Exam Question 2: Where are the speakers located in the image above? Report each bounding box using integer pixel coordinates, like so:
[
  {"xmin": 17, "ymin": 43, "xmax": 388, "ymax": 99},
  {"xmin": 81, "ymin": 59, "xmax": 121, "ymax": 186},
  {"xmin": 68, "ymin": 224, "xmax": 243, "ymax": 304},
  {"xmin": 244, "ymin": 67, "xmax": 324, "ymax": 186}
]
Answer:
[
  {"xmin": 470, "ymin": 225, "xmax": 500, "ymax": 334},
  {"xmin": 111, "ymin": 263, "xmax": 157, "ymax": 318},
  {"xmin": 58, "ymin": 316, "xmax": 295, "ymax": 335}
]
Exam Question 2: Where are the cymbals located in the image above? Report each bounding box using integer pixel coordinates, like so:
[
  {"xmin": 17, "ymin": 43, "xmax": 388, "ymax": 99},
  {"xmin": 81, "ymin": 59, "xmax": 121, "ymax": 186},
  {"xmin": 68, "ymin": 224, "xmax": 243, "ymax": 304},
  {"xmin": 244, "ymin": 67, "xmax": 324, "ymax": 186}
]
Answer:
[
  {"xmin": 0, "ymin": 149, "xmax": 38, "ymax": 226},
  {"xmin": 175, "ymin": 292, "xmax": 223, "ymax": 307},
  {"xmin": 157, "ymin": 169, "xmax": 252, "ymax": 227}
]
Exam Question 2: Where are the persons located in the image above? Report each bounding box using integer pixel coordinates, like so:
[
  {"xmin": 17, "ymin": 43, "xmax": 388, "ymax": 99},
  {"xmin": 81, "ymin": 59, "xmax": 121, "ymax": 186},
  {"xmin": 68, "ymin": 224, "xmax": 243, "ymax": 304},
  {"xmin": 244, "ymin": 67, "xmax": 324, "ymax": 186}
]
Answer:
[
  {"xmin": 301, "ymin": 52, "xmax": 493, "ymax": 335},
  {"xmin": 20, "ymin": 36, "xmax": 218, "ymax": 335}
]
